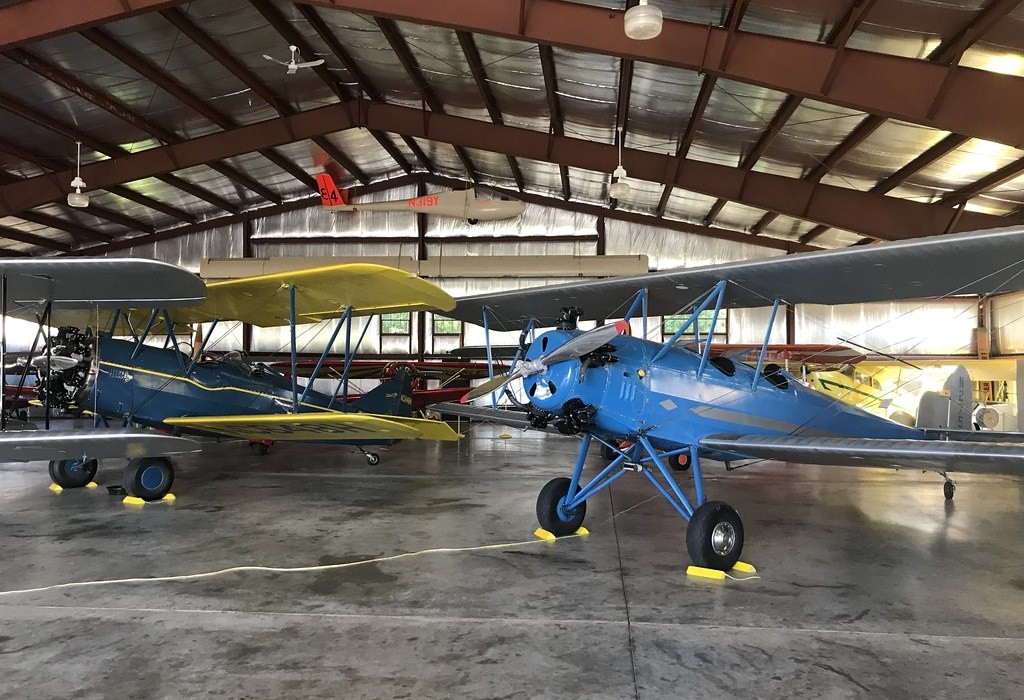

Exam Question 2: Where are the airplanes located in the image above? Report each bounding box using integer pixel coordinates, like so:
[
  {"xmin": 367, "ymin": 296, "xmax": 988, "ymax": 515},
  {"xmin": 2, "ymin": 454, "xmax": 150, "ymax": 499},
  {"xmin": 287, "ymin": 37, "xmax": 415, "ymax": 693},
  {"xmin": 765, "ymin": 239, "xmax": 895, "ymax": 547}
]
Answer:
[
  {"xmin": 2, "ymin": 261, "xmax": 461, "ymax": 502},
  {"xmin": 2, "ymin": 256, "xmax": 208, "ymax": 463},
  {"xmin": 268, "ymin": 355, "xmax": 517, "ymax": 408},
  {"xmin": 804, "ymin": 363, "xmax": 918, "ymax": 420},
  {"xmin": 426, "ymin": 225, "xmax": 1023, "ymax": 572}
]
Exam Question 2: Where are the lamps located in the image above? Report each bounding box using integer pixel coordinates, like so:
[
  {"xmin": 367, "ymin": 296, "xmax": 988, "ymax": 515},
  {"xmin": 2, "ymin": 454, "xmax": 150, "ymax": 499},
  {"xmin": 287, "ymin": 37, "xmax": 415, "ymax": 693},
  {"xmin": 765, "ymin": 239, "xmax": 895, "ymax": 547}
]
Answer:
[
  {"xmin": 67, "ymin": 141, "xmax": 89, "ymax": 207},
  {"xmin": 624, "ymin": 0, "xmax": 664, "ymax": 41},
  {"xmin": 609, "ymin": 126, "xmax": 630, "ymax": 200}
]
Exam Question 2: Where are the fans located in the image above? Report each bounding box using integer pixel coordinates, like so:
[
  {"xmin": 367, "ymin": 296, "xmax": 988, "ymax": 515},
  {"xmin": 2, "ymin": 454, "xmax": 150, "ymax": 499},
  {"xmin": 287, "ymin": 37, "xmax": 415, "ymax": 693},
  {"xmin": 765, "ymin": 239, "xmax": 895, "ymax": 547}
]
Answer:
[{"xmin": 262, "ymin": 45, "xmax": 325, "ymax": 75}]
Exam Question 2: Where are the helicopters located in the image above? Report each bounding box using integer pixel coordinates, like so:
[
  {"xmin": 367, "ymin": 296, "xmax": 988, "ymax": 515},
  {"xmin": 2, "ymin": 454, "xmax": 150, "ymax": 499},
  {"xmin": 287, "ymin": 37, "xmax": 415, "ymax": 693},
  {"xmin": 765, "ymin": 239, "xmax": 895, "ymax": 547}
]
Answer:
[{"xmin": 315, "ymin": 171, "xmax": 527, "ymax": 225}]
